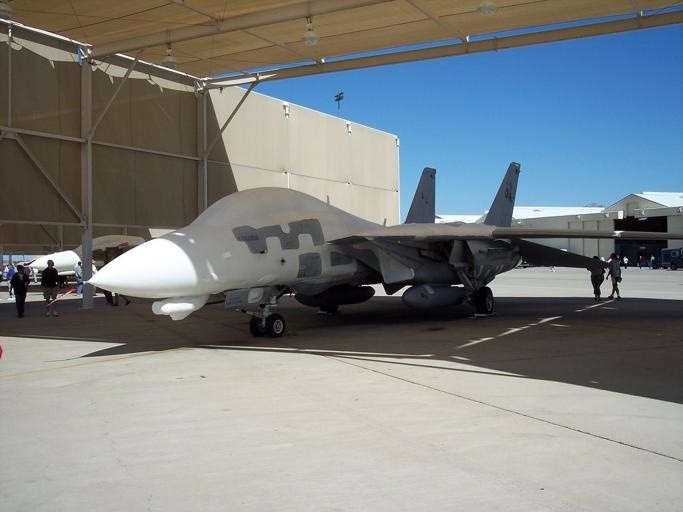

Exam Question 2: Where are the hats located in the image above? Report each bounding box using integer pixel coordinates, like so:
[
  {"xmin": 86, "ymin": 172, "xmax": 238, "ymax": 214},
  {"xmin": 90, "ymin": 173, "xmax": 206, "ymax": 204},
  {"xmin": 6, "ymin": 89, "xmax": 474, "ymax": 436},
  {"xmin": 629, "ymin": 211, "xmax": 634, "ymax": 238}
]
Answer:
[{"xmin": 17, "ymin": 265, "xmax": 25, "ymax": 270}]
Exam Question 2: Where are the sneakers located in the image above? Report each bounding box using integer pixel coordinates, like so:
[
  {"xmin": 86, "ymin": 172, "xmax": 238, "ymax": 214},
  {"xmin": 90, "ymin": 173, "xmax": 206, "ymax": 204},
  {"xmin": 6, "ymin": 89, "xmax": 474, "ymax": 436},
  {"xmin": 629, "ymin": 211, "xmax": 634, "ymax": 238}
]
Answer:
[
  {"xmin": 124, "ymin": 300, "xmax": 131, "ymax": 305},
  {"xmin": 45, "ymin": 311, "xmax": 51, "ymax": 317},
  {"xmin": 53, "ymin": 311, "xmax": 59, "ymax": 317},
  {"xmin": 112, "ymin": 304, "xmax": 119, "ymax": 306}
]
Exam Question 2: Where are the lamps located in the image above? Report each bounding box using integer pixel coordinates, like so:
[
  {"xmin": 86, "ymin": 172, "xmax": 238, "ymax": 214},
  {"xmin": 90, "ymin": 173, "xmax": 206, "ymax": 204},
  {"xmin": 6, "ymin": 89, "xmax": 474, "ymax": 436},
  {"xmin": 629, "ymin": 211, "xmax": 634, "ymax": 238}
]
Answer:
[
  {"xmin": 161, "ymin": 30, "xmax": 176, "ymax": 68},
  {"xmin": 302, "ymin": 1, "xmax": 320, "ymax": 45},
  {"xmin": 479, "ymin": 1, "xmax": 496, "ymax": 16}
]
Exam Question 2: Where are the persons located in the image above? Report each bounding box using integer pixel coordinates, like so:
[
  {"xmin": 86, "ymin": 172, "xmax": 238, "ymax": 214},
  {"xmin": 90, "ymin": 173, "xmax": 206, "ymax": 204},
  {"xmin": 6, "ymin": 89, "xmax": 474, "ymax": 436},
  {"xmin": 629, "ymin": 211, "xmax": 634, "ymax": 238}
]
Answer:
[
  {"xmin": 587, "ymin": 253, "xmax": 622, "ymax": 301},
  {"xmin": 638, "ymin": 256, "xmax": 643, "ymax": 269},
  {"xmin": 0, "ymin": 262, "xmax": 31, "ymax": 318},
  {"xmin": 650, "ymin": 254, "xmax": 655, "ymax": 269},
  {"xmin": 92, "ymin": 259, "xmax": 131, "ymax": 306},
  {"xmin": 41, "ymin": 260, "xmax": 61, "ymax": 318},
  {"xmin": 75, "ymin": 262, "xmax": 83, "ymax": 297},
  {"xmin": 623, "ymin": 256, "xmax": 629, "ymax": 269}
]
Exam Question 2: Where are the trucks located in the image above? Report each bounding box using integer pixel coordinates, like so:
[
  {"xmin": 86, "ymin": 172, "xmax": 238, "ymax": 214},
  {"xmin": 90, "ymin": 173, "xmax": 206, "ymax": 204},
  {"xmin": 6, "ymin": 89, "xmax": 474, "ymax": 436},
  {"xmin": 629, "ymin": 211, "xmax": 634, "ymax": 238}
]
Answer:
[{"xmin": 662, "ymin": 249, "xmax": 682, "ymax": 270}]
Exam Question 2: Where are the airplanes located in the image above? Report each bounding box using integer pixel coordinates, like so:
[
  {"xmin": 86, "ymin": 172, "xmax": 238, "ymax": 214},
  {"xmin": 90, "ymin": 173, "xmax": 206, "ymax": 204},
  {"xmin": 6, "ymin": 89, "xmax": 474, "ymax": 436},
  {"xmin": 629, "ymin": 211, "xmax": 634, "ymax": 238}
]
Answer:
[
  {"xmin": 25, "ymin": 235, "xmax": 147, "ymax": 280},
  {"xmin": 87, "ymin": 162, "xmax": 682, "ymax": 338}
]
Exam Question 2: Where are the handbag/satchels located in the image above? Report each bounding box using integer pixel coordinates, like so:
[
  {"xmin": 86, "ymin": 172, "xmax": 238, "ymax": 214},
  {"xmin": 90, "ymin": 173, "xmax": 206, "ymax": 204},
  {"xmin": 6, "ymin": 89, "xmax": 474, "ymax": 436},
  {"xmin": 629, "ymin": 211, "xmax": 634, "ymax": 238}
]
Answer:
[{"xmin": 615, "ymin": 276, "xmax": 622, "ymax": 283}]
